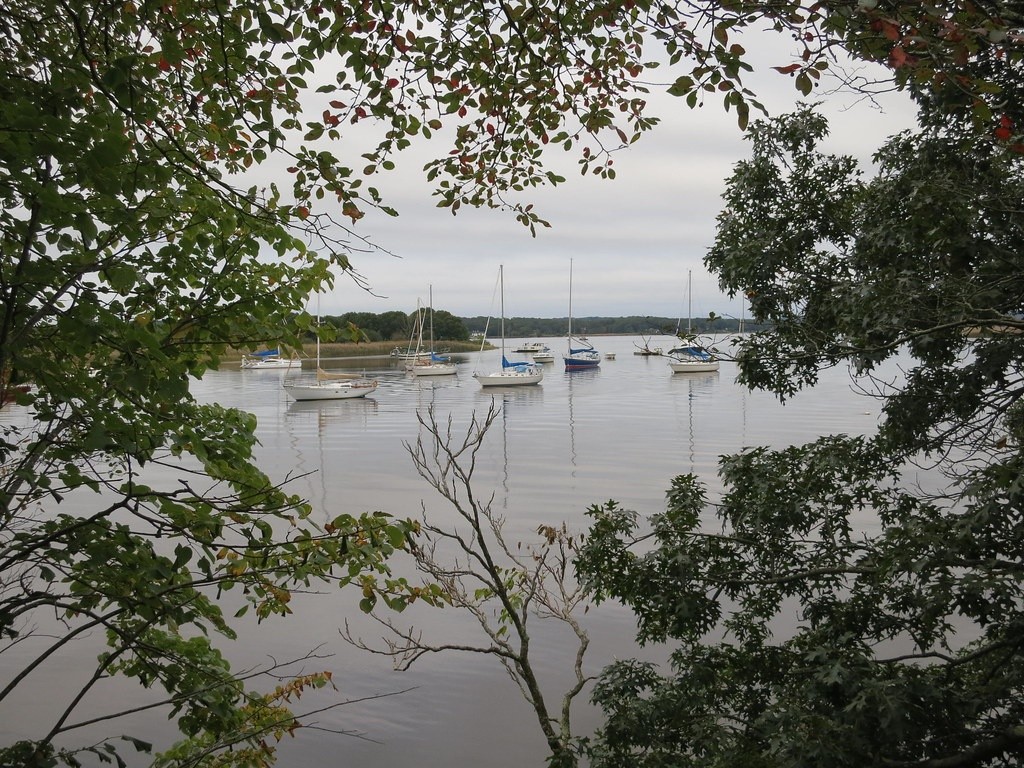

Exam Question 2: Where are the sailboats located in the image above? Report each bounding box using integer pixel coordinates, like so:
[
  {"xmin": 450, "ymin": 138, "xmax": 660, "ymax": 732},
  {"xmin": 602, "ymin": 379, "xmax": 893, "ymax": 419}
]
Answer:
[
  {"xmin": 390, "ymin": 281, "xmax": 459, "ymax": 375},
  {"xmin": 562, "ymin": 256, "xmax": 602, "ymax": 368},
  {"xmin": 280, "ymin": 275, "xmax": 379, "ymax": 401},
  {"xmin": 668, "ymin": 270, "xmax": 721, "ymax": 373}
]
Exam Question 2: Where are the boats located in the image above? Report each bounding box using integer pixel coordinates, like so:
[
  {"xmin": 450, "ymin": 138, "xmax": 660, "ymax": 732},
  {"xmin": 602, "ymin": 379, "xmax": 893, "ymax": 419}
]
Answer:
[
  {"xmin": 471, "ymin": 264, "xmax": 544, "ymax": 387},
  {"xmin": 238, "ymin": 337, "xmax": 302, "ymax": 370},
  {"xmin": 605, "ymin": 353, "xmax": 616, "ymax": 361},
  {"xmin": 511, "ymin": 342, "xmax": 550, "ymax": 353},
  {"xmin": 532, "ymin": 352, "xmax": 554, "ymax": 363}
]
